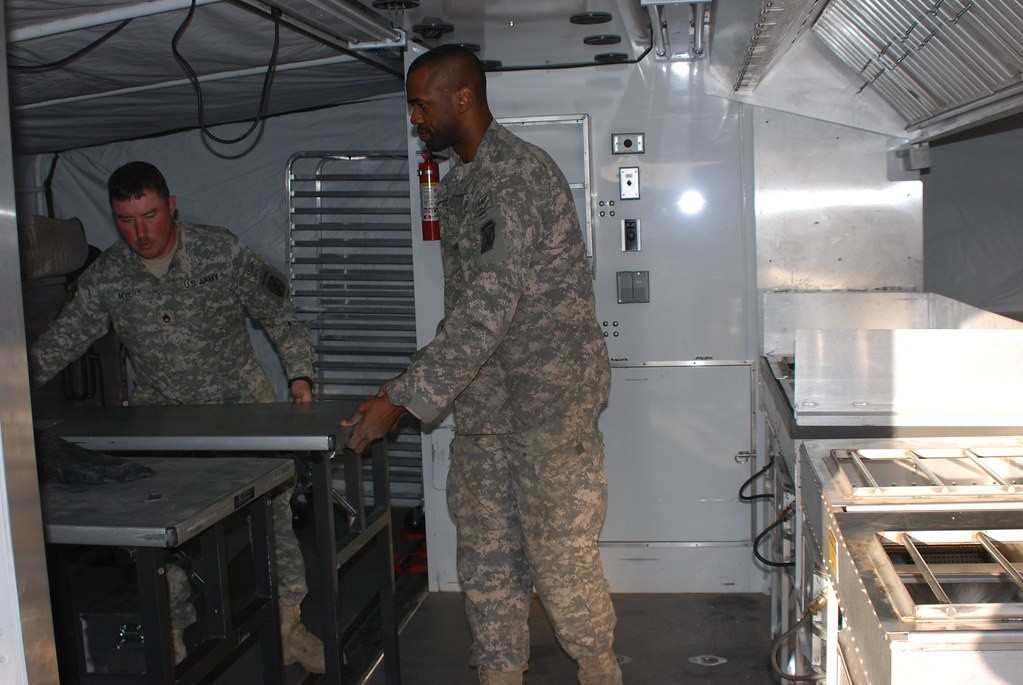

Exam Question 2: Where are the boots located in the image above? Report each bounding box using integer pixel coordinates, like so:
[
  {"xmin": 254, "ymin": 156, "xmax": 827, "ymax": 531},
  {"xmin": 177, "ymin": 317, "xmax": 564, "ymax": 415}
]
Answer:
[
  {"xmin": 477, "ymin": 664, "xmax": 528, "ymax": 685},
  {"xmin": 577, "ymin": 648, "xmax": 622, "ymax": 685},
  {"xmin": 170, "ymin": 629, "xmax": 188, "ymax": 666},
  {"xmin": 279, "ymin": 605, "xmax": 347, "ymax": 672}
]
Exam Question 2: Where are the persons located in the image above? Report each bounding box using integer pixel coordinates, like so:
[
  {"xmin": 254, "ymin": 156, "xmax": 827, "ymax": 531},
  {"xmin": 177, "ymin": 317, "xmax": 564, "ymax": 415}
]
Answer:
[
  {"xmin": 338, "ymin": 43, "xmax": 624, "ymax": 685},
  {"xmin": 27, "ymin": 162, "xmax": 348, "ymax": 673}
]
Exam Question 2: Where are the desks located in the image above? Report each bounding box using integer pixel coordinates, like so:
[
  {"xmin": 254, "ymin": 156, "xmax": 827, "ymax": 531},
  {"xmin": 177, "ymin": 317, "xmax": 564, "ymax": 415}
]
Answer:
[
  {"xmin": 44, "ymin": 402, "xmax": 402, "ymax": 685},
  {"xmin": 25, "ymin": 455, "xmax": 298, "ymax": 685}
]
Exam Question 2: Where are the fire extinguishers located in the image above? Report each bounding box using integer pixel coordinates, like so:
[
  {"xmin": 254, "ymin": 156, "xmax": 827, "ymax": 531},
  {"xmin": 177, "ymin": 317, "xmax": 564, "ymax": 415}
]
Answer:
[{"xmin": 417, "ymin": 150, "xmax": 449, "ymax": 241}]
